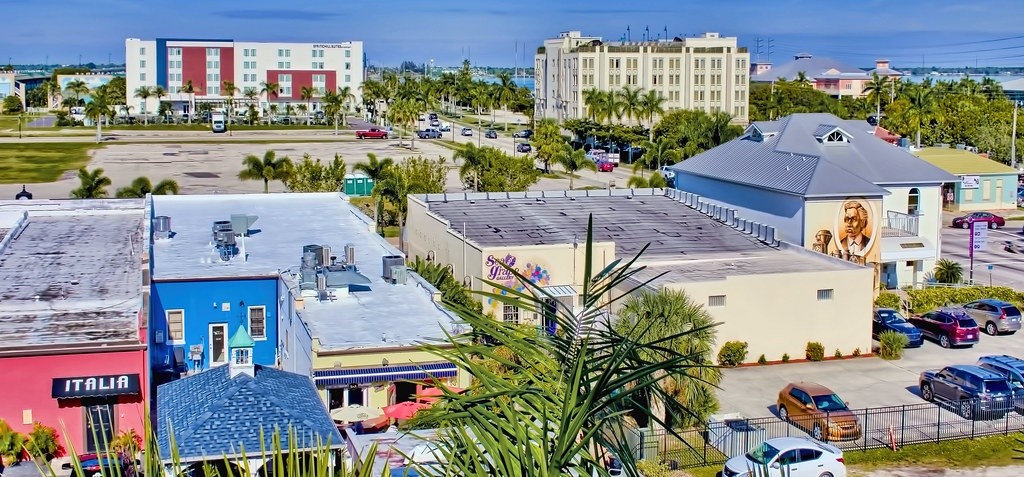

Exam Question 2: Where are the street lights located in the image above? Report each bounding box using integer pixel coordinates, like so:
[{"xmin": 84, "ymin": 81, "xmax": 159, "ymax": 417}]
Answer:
[
  {"xmin": 478, "ymin": 125, "xmax": 481, "ymax": 148},
  {"xmin": 514, "ymin": 133, "xmax": 516, "ymax": 157},
  {"xmin": 452, "ymin": 120, "xmax": 456, "ymax": 142}
]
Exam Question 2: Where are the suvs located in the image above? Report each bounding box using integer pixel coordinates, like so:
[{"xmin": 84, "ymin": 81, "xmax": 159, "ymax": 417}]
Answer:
[
  {"xmin": 976, "ymin": 355, "xmax": 1024, "ymax": 409},
  {"xmin": 920, "ymin": 364, "xmax": 1015, "ymax": 420},
  {"xmin": 909, "ymin": 308, "xmax": 980, "ymax": 349}
]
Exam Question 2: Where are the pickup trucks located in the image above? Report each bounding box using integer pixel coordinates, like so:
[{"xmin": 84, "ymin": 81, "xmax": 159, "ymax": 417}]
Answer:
[
  {"xmin": 356, "ymin": 128, "xmax": 388, "ymax": 139},
  {"xmin": 416, "ymin": 129, "xmax": 443, "ymax": 139}
]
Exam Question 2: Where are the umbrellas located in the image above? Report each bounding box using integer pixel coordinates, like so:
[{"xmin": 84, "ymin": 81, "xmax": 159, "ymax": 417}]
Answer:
[
  {"xmin": 330, "ymin": 404, "xmax": 385, "ymax": 432},
  {"xmin": 382, "ymin": 385, "xmax": 465, "ymax": 419}
]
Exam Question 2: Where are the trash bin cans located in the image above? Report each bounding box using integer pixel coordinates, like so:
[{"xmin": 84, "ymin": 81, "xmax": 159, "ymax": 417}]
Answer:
[{"xmin": 342, "ymin": 174, "xmax": 375, "ymax": 196}]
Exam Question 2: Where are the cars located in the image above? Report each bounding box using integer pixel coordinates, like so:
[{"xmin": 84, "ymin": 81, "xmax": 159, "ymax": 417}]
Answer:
[
  {"xmin": 586, "ymin": 149, "xmax": 614, "ymax": 172},
  {"xmin": 1017, "ymin": 184, "xmax": 1024, "ymax": 208},
  {"xmin": 512, "ymin": 129, "xmax": 532, "ymax": 153},
  {"xmin": 656, "ymin": 165, "xmax": 675, "ymax": 180},
  {"xmin": 872, "ymin": 308, "xmax": 924, "ymax": 347},
  {"xmin": 952, "ymin": 212, "xmax": 1006, "ymax": 230},
  {"xmin": 949, "ymin": 299, "xmax": 1022, "ymax": 336},
  {"xmin": 778, "ymin": 381, "xmax": 863, "ymax": 442},
  {"xmin": 418, "ymin": 113, "xmax": 497, "ymax": 139},
  {"xmin": 722, "ymin": 436, "xmax": 847, "ymax": 477}
]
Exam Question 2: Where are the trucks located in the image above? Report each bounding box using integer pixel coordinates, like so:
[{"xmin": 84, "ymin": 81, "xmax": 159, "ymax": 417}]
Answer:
[
  {"xmin": 211, "ymin": 113, "xmax": 225, "ymax": 132},
  {"xmin": 597, "ymin": 152, "xmax": 619, "ymax": 167}
]
